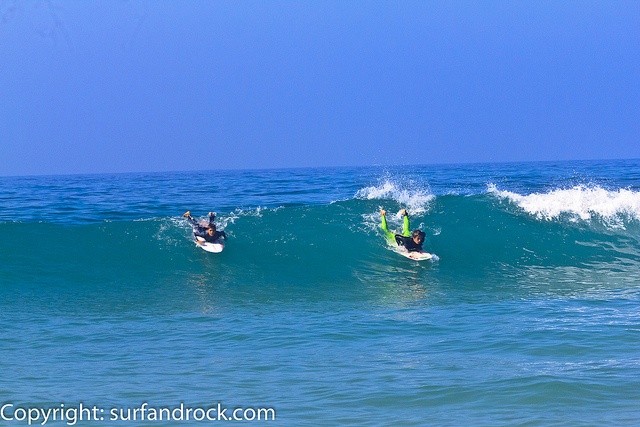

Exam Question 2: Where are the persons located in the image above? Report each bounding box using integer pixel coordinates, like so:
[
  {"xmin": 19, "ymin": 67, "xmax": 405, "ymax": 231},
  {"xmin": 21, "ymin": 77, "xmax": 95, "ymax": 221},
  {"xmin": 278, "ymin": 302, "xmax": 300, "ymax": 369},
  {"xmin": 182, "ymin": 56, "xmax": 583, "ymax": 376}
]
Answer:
[
  {"xmin": 184, "ymin": 210, "xmax": 228, "ymax": 244},
  {"xmin": 380, "ymin": 208, "xmax": 426, "ymax": 254}
]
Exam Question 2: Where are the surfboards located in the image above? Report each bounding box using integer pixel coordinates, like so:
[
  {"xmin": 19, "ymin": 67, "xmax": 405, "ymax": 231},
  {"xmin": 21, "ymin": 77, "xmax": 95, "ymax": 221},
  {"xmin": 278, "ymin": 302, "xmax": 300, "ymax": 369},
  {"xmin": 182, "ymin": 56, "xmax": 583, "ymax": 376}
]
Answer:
[
  {"xmin": 395, "ymin": 244, "xmax": 432, "ymax": 260},
  {"xmin": 193, "ymin": 218, "xmax": 224, "ymax": 253}
]
